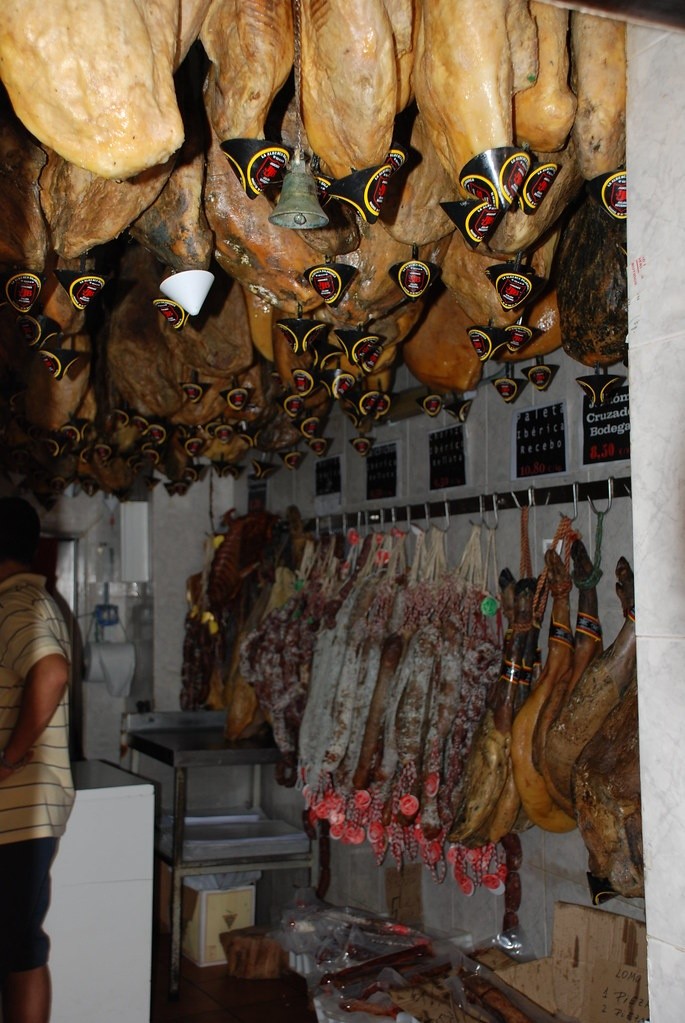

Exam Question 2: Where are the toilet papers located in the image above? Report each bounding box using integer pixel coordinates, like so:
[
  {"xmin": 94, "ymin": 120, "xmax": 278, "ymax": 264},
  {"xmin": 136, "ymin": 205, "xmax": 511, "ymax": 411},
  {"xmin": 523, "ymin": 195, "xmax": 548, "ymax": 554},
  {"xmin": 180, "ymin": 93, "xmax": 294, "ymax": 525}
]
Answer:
[{"xmin": 82, "ymin": 640, "xmax": 137, "ymax": 699}]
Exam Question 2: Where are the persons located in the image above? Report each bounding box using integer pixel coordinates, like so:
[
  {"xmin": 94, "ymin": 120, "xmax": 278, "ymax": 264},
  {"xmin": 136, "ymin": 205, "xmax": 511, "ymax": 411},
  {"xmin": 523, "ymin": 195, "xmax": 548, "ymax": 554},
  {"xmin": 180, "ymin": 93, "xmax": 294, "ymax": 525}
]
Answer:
[{"xmin": 0, "ymin": 495, "xmax": 75, "ymax": 1023}]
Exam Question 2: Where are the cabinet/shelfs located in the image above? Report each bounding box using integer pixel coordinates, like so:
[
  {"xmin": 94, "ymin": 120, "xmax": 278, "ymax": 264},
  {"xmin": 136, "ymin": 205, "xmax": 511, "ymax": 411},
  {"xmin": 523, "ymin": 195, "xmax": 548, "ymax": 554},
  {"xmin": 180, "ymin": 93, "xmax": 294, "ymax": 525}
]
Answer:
[{"xmin": 131, "ymin": 725, "xmax": 322, "ymax": 994}]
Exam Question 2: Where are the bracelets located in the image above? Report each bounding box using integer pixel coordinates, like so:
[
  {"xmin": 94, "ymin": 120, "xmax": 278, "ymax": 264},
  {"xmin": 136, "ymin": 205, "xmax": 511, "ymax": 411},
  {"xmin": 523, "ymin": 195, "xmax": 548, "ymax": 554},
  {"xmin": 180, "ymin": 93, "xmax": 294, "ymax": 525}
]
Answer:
[{"xmin": 0, "ymin": 748, "xmax": 28, "ymax": 769}]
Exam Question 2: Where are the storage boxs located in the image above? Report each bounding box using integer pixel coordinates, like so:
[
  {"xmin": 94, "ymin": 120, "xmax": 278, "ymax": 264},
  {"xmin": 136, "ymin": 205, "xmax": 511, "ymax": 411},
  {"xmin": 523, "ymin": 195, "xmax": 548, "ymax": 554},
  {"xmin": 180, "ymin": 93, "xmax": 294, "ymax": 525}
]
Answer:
[{"xmin": 181, "ymin": 883, "xmax": 256, "ymax": 967}]
